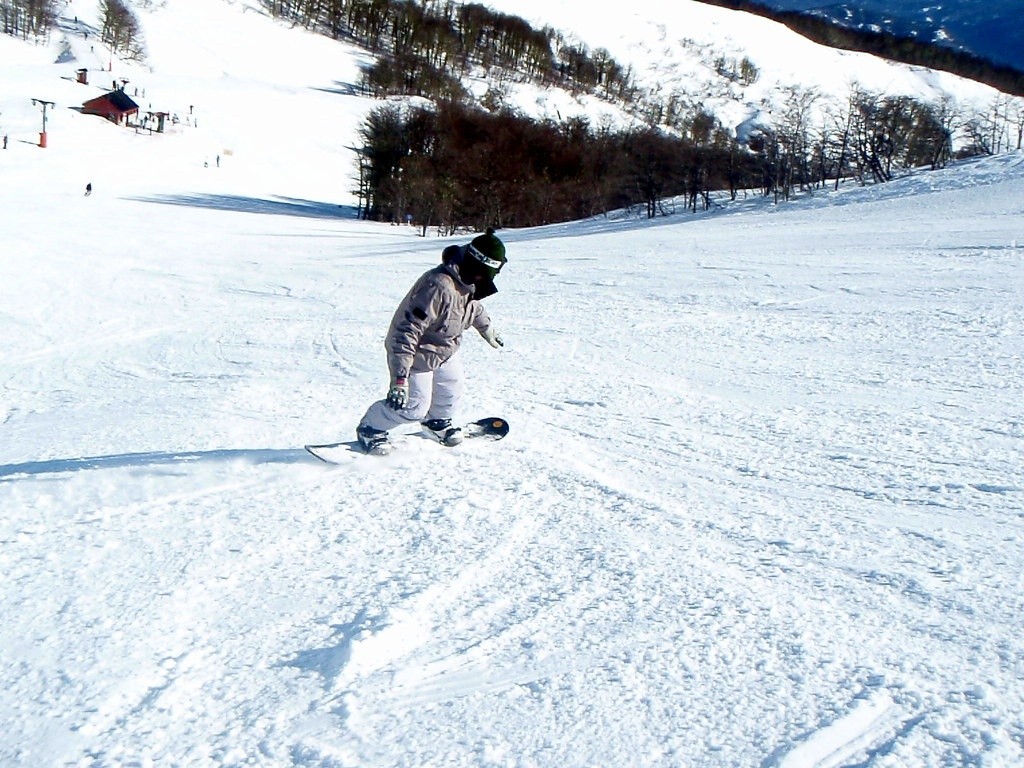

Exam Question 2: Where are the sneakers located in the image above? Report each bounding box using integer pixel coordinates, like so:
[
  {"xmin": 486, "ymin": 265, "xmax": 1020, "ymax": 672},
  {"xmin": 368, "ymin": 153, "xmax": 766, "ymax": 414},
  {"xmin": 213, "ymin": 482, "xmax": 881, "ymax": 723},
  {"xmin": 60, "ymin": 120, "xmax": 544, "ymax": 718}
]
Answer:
[
  {"xmin": 355, "ymin": 417, "xmax": 392, "ymax": 454},
  {"xmin": 419, "ymin": 411, "xmax": 463, "ymax": 447}
]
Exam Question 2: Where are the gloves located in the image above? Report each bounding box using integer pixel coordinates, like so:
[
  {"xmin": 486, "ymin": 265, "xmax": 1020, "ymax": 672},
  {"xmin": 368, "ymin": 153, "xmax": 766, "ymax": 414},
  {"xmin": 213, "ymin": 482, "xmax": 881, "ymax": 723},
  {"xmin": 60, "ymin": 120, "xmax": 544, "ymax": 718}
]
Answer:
[
  {"xmin": 385, "ymin": 376, "xmax": 410, "ymax": 410},
  {"xmin": 479, "ymin": 327, "xmax": 505, "ymax": 349}
]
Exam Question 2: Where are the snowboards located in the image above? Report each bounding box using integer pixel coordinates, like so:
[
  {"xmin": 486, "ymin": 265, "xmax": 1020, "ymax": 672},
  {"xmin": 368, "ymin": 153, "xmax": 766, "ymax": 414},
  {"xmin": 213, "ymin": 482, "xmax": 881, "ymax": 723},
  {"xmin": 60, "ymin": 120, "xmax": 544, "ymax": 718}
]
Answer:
[{"xmin": 303, "ymin": 416, "xmax": 510, "ymax": 466}]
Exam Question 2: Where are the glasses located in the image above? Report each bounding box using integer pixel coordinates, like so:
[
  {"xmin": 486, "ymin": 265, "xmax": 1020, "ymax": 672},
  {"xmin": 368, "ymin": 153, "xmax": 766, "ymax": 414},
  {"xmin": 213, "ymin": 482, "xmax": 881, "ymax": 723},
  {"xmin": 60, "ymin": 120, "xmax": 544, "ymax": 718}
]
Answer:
[{"xmin": 496, "ymin": 257, "xmax": 507, "ymax": 274}]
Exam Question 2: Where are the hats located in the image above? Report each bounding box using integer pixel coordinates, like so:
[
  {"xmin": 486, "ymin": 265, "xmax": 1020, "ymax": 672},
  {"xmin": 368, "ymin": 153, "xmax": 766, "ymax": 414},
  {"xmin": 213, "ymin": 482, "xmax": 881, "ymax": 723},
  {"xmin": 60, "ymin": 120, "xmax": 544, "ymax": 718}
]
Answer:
[{"xmin": 465, "ymin": 226, "xmax": 507, "ymax": 280}]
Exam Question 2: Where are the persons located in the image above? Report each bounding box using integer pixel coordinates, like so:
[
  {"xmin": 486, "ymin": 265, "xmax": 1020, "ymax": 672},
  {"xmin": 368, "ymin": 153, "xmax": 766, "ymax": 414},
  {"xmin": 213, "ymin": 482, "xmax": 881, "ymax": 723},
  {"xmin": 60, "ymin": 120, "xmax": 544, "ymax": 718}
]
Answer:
[
  {"xmin": 86, "ymin": 183, "xmax": 91, "ymax": 194},
  {"xmin": 3, "ymin": 135, "xmax": 7, "ymax": 149},
  {"xmin": 357, "ymin": 226, "xmax": 507, "ymax": 456}
]
